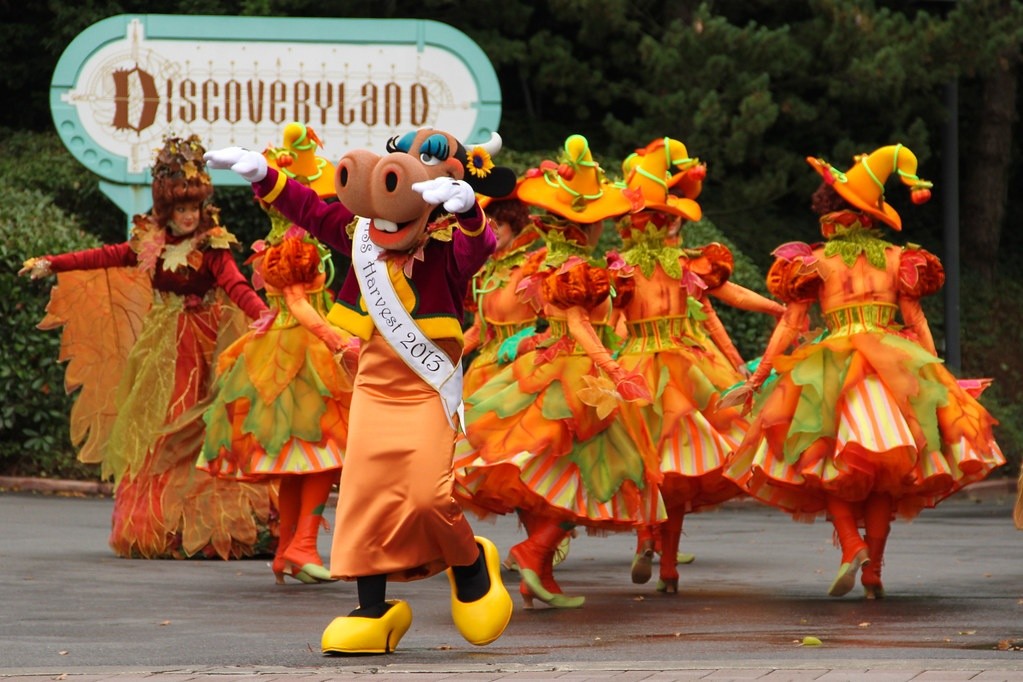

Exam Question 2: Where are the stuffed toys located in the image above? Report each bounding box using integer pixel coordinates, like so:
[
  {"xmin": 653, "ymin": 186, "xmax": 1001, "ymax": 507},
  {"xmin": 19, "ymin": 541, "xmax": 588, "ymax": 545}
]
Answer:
[{"xmin": 204, "ymin": 127, "xmax": 516, "ymax": 654}]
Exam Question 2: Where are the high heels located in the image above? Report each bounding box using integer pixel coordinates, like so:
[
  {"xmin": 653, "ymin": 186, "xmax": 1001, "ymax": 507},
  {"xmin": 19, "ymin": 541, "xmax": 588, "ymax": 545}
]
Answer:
[
  {"xmin": 502, "ymin": 543, "xmax": 555, "ymax": 602},
  {"xmin": 826, "ymin": 549, "xmax": 870, "ymax": 599},
  {"xmin": 281, "ymin": 546, "xmax": 338, "ymax": 581},
  {"xmin": 518, "ymin": 577, "xmax": 586, "ymax": 610},
  {"xmin": 658, "ymin": 547, "xmax": 694, "ymax": 564},
  {"xmin": 271, "ymin": 557, "xmax": 321, "ymax": 586},
  {"xmin": 654, "ymin": 574, "xmax": 680, "ymax": 594},
  {"xmin": 860, "ymin": 573, "xmax": 886, "ymax": 601},
  {"xmin": 631, "ymin": 540, "xmax": 654, "ymax": 586},
  {"xmin": 551, "ymin": 528, "xmax": 578, "ymax": 566}
]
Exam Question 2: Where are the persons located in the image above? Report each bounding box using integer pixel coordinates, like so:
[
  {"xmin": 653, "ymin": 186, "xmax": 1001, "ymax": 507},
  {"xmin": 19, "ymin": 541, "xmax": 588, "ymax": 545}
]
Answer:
[
  {"xmin": 589, "ymin": 139, "xmax": 750, "ymax": 594},
  {"xmin": 658, "ymin": 210, "xmax": 788, "ymax": 566},
  {"xmin": 17, "ymin": 134, "xmax": 284, "ymax": 560},
  {"xmin": 194, "ymin": 122, "xmax": 355, "ymax": 586},
  {"xmin": 450, "ymin": 134, "xmax": 668, "ymax": 608},
  {"xmin": 456, "ymin": 199, "xmax": 570, "ymax": 566},
  {"xmin": 713, "ymin": 145, "xmax": 1006, "ymax": 599}
]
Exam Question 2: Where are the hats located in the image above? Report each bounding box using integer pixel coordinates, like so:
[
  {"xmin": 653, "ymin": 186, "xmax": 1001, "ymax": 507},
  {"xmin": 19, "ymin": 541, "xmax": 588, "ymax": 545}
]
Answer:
[
  {"xmin": 259, "ymin": 120, "xmax": 341, "ymax": 200},
  {"xmin": 620, "ymin": 149, "xmax": 703, "ymax": 226},
  {"xmin": 516, "ymin": 133, "xmax": 633, "ymax": 227},
  {"xmin": 805, "ymin": 143, "xmax": 933, "ymax": 234}
]
